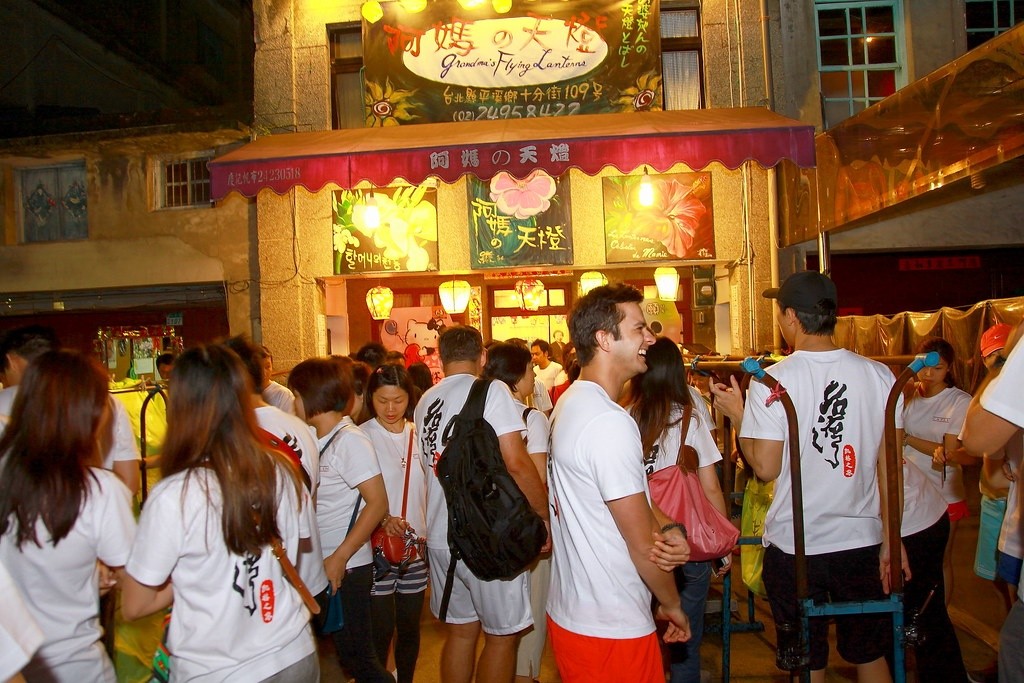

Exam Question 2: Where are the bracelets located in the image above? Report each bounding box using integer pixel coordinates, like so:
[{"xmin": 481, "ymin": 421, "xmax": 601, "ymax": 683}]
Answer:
[
  {"xmin": 902, "ymin": 433, "xmax": 909, "ymax": 447},
  {"xmin": 662, "ymin": 523, "xmax": 688, "ymax": 539}
]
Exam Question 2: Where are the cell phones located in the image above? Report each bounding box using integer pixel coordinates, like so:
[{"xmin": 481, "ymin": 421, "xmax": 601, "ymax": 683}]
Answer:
[{"xmin": 711, "ymin": 555, "xmax": 729, "ymax": 576}]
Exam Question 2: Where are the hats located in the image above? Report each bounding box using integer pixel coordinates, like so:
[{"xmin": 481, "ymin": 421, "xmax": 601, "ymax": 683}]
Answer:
[
  {"xmin": 762, "ymin": 271, "xmax": 838, "ymax": 317},
  {"xmin": 980, "ymin": 323, "xmax": 1014, "ymax": 357}
]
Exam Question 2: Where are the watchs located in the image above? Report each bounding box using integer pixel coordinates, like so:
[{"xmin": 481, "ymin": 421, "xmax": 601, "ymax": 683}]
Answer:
[{"xmin": 380, "ymin": 512, "xmax": 391, "ymax": 526}]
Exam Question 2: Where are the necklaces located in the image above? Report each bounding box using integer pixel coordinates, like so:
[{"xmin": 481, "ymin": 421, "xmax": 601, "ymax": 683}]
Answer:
[{"xmin": 378, "ymin": 416, "xmax": 407, "ymax": 468}]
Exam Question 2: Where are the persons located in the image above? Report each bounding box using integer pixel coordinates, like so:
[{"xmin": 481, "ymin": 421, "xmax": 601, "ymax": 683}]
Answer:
[{"xmin": 0, "ymin": 272, "xmax": 1024, "ymax": 683}]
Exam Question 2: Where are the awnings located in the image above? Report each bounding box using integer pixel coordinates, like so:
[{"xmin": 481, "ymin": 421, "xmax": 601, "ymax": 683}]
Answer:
[{"xmin": 208, "ymin": 104, "xmax": 818, "ymax": 205}]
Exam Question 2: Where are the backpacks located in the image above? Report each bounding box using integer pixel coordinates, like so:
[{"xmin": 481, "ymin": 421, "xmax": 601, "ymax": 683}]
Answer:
[{"xmin": 436, "ymin": 378, "xmax": 548, "ymax": 623}]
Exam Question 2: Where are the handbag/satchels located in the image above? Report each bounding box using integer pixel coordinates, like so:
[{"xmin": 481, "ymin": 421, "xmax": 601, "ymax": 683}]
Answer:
[
  {"xmin": 370, "ymin": 551, "xmax": 391, "ymax": 580},
  {"xmin": 369, "ymin": 522, "xmax": 418, "ymax": 564},
  {"xmin": 323, "ymin": 583, "xmax": 345, "ymax": 635},
  {"xmin": 644, "ymin": 398, "xmax": 741, "ymax": 563},
  {"xmin": 740, "ymin": 465, "xmax": 780, "ymax": 600}
]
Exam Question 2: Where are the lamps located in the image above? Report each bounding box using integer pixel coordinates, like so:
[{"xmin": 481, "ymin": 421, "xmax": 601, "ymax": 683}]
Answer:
[
  {"xmin": 515, "ymin": 280, "xmax": 545, "ymax": 312},
  {"xmin": 580, "ymin": 270, "xmax": 609, "ymax": 296},
  {"xmin": 438, "ymin": 275, "xmax": 470, "ymax": 314},
  {"xmin": 654, "ymin": 267, "xmax": 680, "ymax": 301},
  {"xmin": 367, "ymin": 280, "xmax": 393, "ymax": 321}
]
